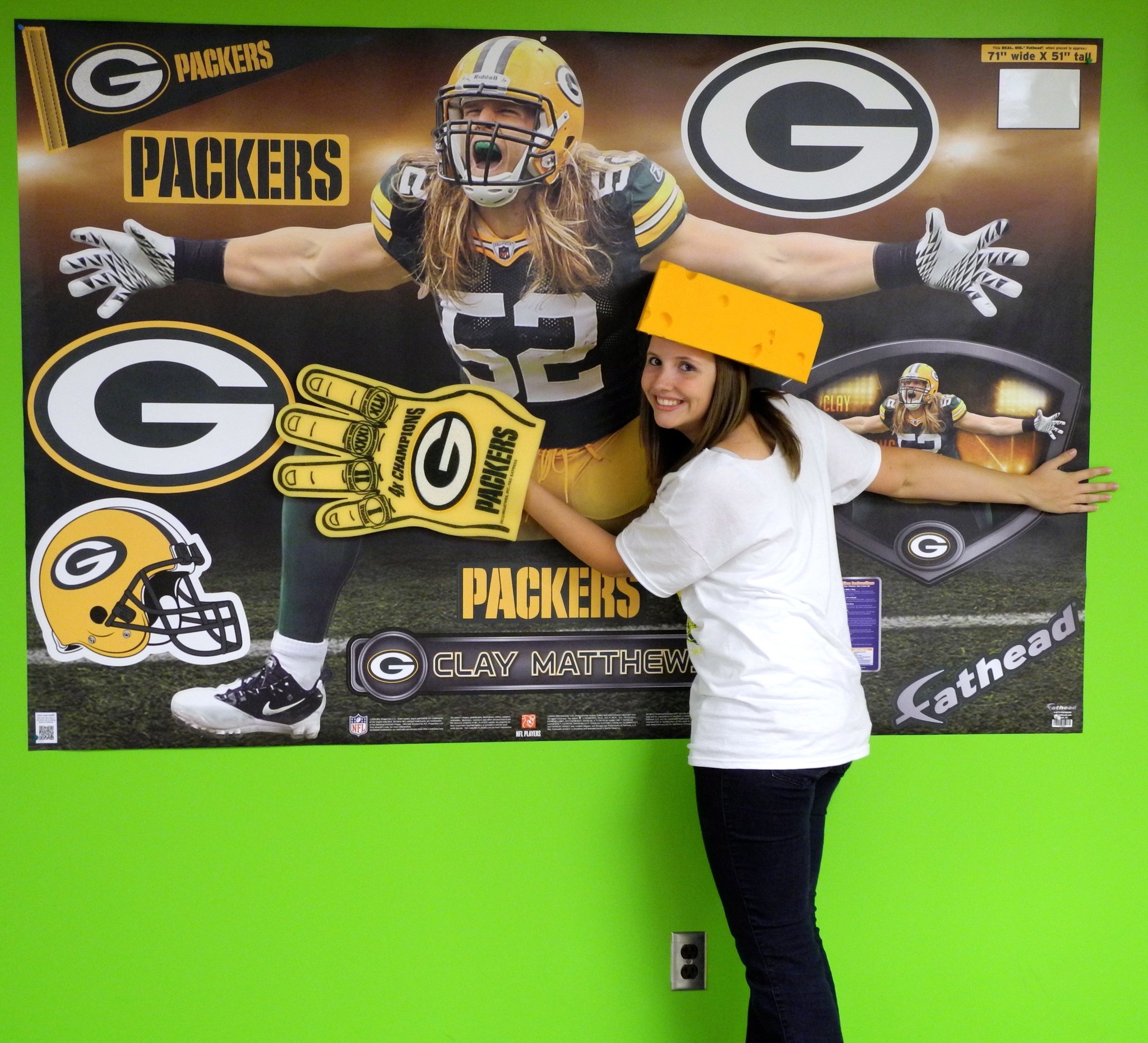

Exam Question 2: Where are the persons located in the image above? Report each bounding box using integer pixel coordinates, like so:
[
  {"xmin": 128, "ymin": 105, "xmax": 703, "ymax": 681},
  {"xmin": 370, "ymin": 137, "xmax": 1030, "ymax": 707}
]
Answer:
[
  {"xmin": 64, "ymin": 37, "xmax": 1030, "ymax": 741},
  {"xmin": 836, "ymin": 362, "xmax": 1067, "ymax": 461},
  {"xmin": 517, "ymin": 333, "xmax": 1118, "ymax": 1043}
]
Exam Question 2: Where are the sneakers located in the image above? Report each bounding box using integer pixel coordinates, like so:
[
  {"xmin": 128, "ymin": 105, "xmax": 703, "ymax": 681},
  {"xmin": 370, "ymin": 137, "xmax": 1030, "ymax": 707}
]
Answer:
[{"xmin": 170, "ymin": 655, "xmax": 325, "ymax": 740}]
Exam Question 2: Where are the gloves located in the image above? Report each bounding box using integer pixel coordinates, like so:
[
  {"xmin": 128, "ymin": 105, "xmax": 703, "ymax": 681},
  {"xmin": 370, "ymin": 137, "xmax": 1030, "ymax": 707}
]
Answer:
[
  {"xmin": 273, "ymin": 364, "xmax": 547, "ymax": 542},
  {"xmin": 1022, "ymin": 409, "xmax": 1065, "ymax": 440},
  {"xmin": 872, "ymin": 207, "xmax": 1030, "ymax": 318},
  {"xmin": 59, "ymin": 216, "xmax": 228, "ymax": 318}
]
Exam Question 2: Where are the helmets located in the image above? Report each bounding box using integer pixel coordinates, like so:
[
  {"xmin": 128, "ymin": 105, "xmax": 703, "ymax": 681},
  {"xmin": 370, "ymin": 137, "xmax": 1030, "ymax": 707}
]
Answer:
[
  {"xmin": 429, "ymin": 35, "xmax": 584, "ymax": 207},
  {"xmin": 897, "ymin": 363, "xmax": 939, "ymax": 410}
]
